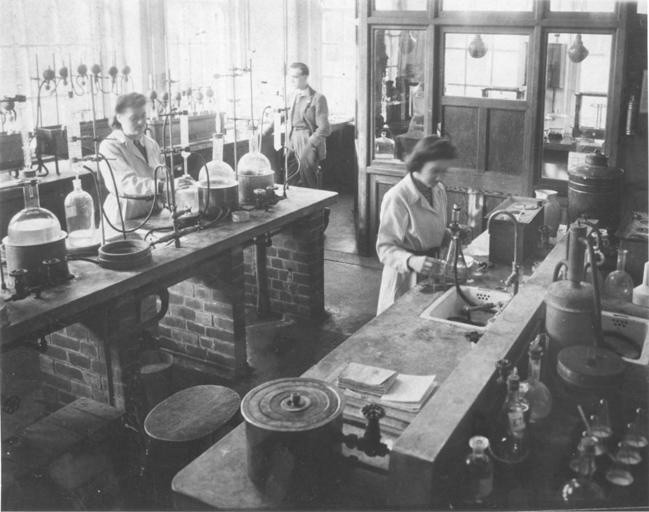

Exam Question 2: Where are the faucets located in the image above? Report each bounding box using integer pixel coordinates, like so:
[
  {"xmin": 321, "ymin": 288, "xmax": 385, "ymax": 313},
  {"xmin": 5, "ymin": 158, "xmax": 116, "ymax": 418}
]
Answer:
[{"xmin": 487, "ymin": 210, "xmax": 519, "ymax": 262}]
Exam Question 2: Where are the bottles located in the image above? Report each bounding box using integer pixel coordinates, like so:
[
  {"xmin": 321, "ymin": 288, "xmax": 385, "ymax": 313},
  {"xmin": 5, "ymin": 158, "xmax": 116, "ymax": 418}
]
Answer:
[
  {"xmin": 63, "ymin": 178, "xmax": 96, "ymax": 247},
  {"xmin": 236, "ymin": 134, "xmax": 271, "ymax": 175},
  {"xmin": 198, "ymin": 133, "xmax": 236, "ymax": 189},
  {"xmin": 602, "ymin": 249, "xmax": 633, "ymax": 304},
  {"xmin": 456, "ymin": 331, "xmax": 649, "ymax": 512},
  {"xmin": 172, "ymin": 173, "xmax": 200, "ymax": 219},
  {"xmin": 408, "ymin": 81, "xmax": 425, "ymax": 117},
  {"xmin": 374, "ymin": 131, "xmax": 395, "ymax": 160},
  {"xmin": 7, "ymin": 169, "xmax": 62, "ymax": 246}
]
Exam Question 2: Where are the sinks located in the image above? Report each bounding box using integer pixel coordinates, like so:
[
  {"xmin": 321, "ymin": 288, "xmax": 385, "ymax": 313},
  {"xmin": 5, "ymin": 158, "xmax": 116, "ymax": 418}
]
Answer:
[{"xmin": 418, "ymin": 285, "xmax": 511, "ymax": 331}]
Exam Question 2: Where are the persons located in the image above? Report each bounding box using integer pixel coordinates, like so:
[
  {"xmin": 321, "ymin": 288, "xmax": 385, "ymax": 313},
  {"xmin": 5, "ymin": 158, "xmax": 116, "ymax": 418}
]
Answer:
[
  {"xmin": 279, "ymin": 61, "xmax": 331, "ymax": 233},
  {"xmin": 376, "ymin": 134, "xmax": 471, "ymax": 316},
  {"xmin": 95, "ymin": 92, "xmax": 168, "ymax": 225}
]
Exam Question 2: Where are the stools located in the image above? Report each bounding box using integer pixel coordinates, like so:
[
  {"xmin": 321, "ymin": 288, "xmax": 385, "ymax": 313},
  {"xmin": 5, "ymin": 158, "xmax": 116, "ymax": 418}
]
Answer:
[{"xmin": 144, "ymin": 382, "xmax": 242, "ymax": 509}]
